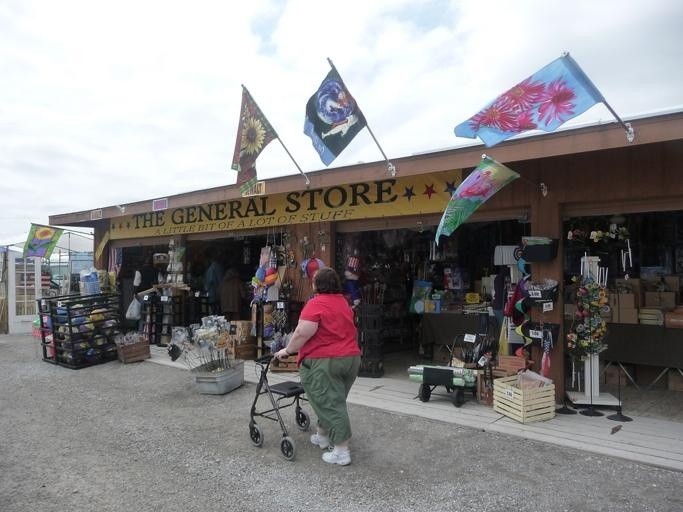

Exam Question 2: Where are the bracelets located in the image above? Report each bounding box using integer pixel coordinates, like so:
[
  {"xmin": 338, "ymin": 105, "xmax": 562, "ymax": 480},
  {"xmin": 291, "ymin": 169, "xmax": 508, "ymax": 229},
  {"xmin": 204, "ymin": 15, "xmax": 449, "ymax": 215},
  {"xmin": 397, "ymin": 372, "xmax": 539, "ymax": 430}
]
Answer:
[{"xmin": 284, "ymin": 348, "xmax": 292, "ymax": 357}]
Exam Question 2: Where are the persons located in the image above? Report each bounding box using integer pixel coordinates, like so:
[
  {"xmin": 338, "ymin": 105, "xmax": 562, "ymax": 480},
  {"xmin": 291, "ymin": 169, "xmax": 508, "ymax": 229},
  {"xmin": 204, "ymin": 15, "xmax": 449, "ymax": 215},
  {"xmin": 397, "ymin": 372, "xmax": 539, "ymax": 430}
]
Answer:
[
  {"xmin": 131, "ymin": 251, "xmax": 246, "ymax": 332},
  {"xmin": 275, "ymin": 266, "xmax": 366, "ymax": 468}
]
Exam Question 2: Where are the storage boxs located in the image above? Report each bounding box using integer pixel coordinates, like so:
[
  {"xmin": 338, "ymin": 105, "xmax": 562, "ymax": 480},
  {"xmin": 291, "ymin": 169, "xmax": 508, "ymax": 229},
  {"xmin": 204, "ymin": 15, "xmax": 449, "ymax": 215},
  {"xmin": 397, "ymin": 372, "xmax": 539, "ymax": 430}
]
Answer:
[
  {"xmin": 187, "ymin": 360, "xmax": 244, "ymax": 395},
  {"xmin": 492, "ymin": 373, "xmax": 556, "ymax": 421},
  {"xmin": 523, "ymin": 239, "xmax": 559, "ymax": 262},
  {"xmin": 116, "ymin": 336, "xmax": 150, "ymax": 363},
  {"xmin": 423, "ymin": 273, "xmax": 682, "ymax": 391}
]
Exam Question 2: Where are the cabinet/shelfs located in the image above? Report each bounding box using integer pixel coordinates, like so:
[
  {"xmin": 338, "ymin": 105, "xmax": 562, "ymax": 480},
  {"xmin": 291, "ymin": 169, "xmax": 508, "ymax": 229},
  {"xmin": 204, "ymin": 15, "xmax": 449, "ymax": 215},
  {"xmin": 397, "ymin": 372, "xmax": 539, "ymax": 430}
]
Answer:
[{"xmin": 138, "ymin": 290, "xmax": 210, "ymax": 349}]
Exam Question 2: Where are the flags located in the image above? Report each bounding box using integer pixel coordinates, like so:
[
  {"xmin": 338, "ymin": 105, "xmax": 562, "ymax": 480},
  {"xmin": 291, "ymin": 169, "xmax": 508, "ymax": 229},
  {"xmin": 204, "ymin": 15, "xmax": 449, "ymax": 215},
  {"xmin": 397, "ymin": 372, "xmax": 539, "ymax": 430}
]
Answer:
[
  {"xmin": 451, "ymin": 49, "xmax": 607, "ymax": 151},
  {"xmin": 21, "ymin": 222, "xmax": 65, "ymax": 261},
  {"xmin": 230, "ymin": 86, "xmax": 279, "ymax": 195},
  {"xmin": 433, "ymin": 152, "xmax": 523, "ymax": 248},
  {"xmin": 300, "ymin": 68, "xmax": 370, "ymax": 168}
]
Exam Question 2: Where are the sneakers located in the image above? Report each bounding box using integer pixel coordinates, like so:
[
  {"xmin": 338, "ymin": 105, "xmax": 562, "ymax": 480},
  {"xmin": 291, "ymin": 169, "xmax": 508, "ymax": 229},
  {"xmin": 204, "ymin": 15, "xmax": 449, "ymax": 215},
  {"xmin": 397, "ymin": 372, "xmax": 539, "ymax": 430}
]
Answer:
[
  {"xmin": 322, "ymin": 451, "xmax": 352, "ymax": 466},
  {"xmin": 311, "ymin": 434, "xmax": 330, "ymax": 450}
]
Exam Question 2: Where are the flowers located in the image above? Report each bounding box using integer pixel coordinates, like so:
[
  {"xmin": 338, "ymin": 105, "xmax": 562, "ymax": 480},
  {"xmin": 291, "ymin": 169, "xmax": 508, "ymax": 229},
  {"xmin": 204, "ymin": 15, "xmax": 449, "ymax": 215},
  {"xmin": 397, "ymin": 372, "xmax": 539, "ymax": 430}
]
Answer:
[{"xmin": 566, "ymin": 222, "xmax": 629, "ymax": 244}]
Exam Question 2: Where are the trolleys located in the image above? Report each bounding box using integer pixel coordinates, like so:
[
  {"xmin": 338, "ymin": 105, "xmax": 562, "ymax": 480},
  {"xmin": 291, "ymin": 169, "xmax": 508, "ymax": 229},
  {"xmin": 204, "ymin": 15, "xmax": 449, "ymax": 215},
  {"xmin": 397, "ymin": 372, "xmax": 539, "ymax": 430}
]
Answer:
[
  {"xmin": 417, "ymin": 331, "xmax": 482, "ymax": 408},
  {"xmin": 248, "ymin": 353, "xmax": 310, "ymax": 462}
]
